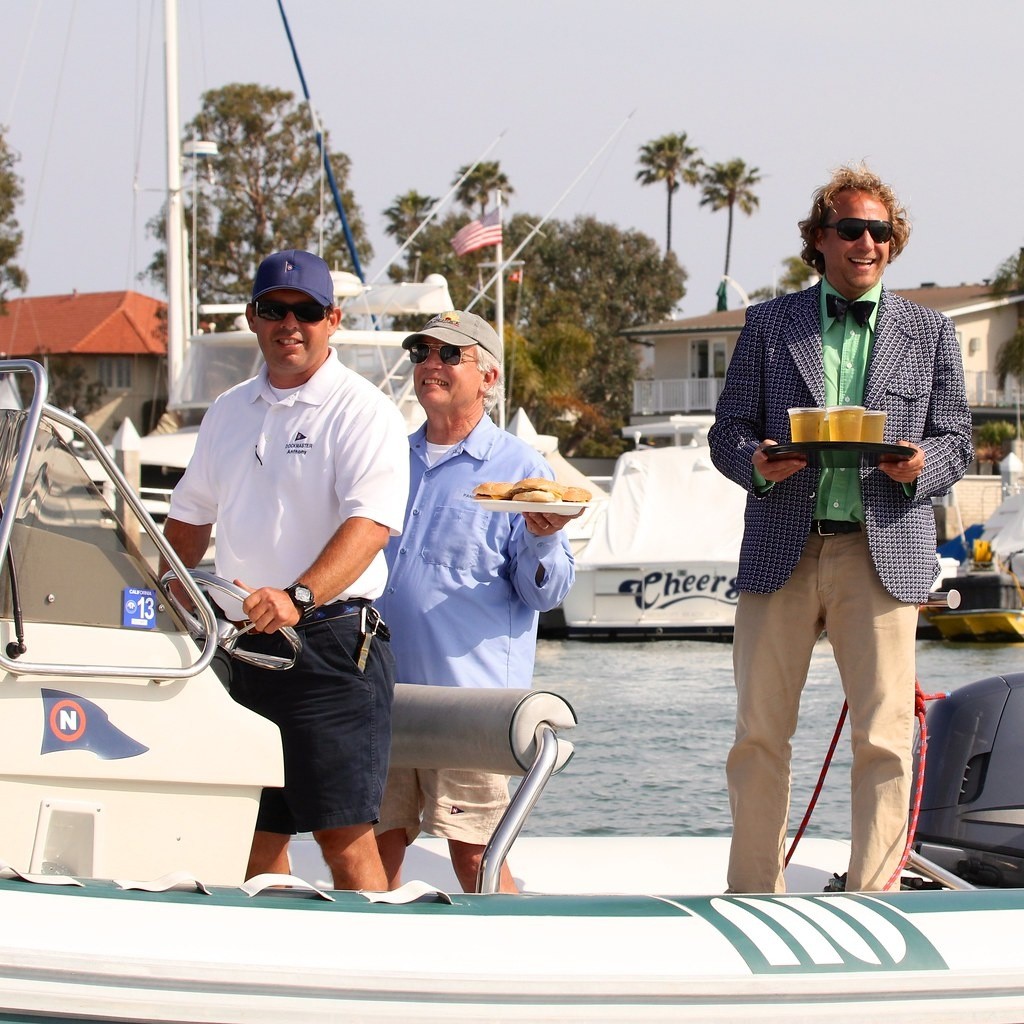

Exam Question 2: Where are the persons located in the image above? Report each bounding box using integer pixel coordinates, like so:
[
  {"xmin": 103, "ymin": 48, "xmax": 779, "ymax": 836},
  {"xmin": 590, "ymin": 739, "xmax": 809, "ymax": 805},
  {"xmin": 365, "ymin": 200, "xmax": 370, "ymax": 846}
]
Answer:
[
  {"xmin": 445, "ymin": 349, "xmax": 460, "ymax": 365},
  {"xmin": 707, "ymin": 154, "xmax": 975, "ymax": 893},
  {"xmin": 158, "ymin": 249, "xmax": 410, "ymax": 892},
  {"xmin": 358, "ymin": 310, "xmax": 585, "ymax": 894}
]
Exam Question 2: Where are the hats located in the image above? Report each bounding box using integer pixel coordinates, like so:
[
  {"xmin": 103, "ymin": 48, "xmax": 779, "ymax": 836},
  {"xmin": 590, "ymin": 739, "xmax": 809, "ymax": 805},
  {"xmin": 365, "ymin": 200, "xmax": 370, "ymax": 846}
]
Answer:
[
  {"xmin": 251, "ymin": 250, "xmax": 335, "ymax": 306},
  {"xmin": 402, "ymin": 307, "xmax": 503, "ymax": 365}
]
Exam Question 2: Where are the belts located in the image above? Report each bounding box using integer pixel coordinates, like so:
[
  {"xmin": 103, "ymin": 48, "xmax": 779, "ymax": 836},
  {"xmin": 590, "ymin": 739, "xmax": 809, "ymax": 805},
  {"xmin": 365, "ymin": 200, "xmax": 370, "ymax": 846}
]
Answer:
[
  {"xmin": 229, "ymin": 597, "xmax": 362, "ymax": 636},
  {"xmin": 809, "ymin": 519, "xmax": 860, "ymax": 535}
]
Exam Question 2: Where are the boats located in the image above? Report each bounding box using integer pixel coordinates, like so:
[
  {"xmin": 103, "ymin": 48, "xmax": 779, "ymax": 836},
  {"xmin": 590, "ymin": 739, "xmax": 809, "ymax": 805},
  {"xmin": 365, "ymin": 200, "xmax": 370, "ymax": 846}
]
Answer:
[
  {"xmin": 0, "ymin": 355, "xmax": 1024, "ymax": 1024},
  {"xmin": 559, "ymin": 415, "xmax": 1024, "ymax": 641}
]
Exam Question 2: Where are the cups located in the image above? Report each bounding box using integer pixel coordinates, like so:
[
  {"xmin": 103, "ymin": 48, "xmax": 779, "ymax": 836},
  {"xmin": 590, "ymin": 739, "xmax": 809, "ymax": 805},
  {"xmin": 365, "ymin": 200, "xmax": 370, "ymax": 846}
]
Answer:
[
  {"xmin": 827, "ymin": 405, "xmax": 866, "ymax": 441},
  {"xmin": 861, "ymin": 410, "xmax": 887, "ymax": 443},
  {"xmin": 822, "ymin": 408, "xmax": 830, "ymax": 442},
  {"xmin": 788, "ymin": 407, "xmax": 825, "ymax": 444}
]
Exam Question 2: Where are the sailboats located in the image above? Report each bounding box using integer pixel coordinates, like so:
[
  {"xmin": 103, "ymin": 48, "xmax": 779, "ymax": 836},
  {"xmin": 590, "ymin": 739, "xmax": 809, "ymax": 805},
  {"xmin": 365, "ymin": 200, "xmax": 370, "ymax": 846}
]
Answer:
[{"xmin": 0, "ymin": 0, "xmax": 616, "ymax": 601}]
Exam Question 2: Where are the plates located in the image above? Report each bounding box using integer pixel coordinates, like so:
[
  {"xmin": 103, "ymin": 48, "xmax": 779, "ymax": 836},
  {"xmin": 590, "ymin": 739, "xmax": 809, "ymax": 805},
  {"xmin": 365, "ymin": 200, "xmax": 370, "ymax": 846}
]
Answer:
[{"xmin": 473, "ymin": 500, "xmax": 589, "ymax": 516}]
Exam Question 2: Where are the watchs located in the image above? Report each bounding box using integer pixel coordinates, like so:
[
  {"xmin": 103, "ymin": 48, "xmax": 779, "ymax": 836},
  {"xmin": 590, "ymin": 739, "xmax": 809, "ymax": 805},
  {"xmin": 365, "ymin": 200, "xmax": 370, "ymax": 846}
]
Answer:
[{"xmin": 284, "ymin": 582, "xmax": 316, "ymax": 621}]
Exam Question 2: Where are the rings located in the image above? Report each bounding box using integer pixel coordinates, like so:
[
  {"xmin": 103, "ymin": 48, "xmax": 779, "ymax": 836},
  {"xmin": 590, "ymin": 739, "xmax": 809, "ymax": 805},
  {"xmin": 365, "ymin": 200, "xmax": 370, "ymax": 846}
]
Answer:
[{"xmin": 543, "ymin": 525, "xmax": 549, "ymax": 530}]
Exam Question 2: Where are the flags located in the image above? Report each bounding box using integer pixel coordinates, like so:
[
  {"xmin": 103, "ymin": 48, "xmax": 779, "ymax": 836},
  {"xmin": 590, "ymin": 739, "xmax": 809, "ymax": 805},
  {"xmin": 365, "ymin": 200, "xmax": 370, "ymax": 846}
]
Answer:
[{"xmin": 450, "ymin": 207, "xmax": 503, "ymax": 256}]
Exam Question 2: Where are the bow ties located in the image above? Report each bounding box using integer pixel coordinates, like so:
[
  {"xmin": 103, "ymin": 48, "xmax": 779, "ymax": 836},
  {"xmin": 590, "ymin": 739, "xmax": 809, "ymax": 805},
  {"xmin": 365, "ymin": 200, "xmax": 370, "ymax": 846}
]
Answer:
[{"xmin": 826, "ymin": 292, "xmax": 877, "ymax": 329}]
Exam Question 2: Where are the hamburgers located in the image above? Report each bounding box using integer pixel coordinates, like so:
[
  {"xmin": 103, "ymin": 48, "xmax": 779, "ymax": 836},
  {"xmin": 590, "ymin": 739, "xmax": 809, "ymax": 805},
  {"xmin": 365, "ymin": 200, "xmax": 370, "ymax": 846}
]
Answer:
[{"xmin": 471, "ymin": 477, "xmax": 593, "ymax": 503}]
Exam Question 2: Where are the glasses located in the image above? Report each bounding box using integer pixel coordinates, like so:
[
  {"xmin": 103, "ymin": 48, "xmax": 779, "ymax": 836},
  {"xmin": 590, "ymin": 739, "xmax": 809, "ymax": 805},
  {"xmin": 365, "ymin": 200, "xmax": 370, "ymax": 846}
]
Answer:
[
  {"xmin": 253, "ymin": 301, "xmax": 331, "ymax": 324},
  {"xmin": 821, "ymin": 216, "xmax": 895, "ymax": 245},
  {"xmin": 406, "ymin": 339, "xmax": 484, "ymax": 367}
]
